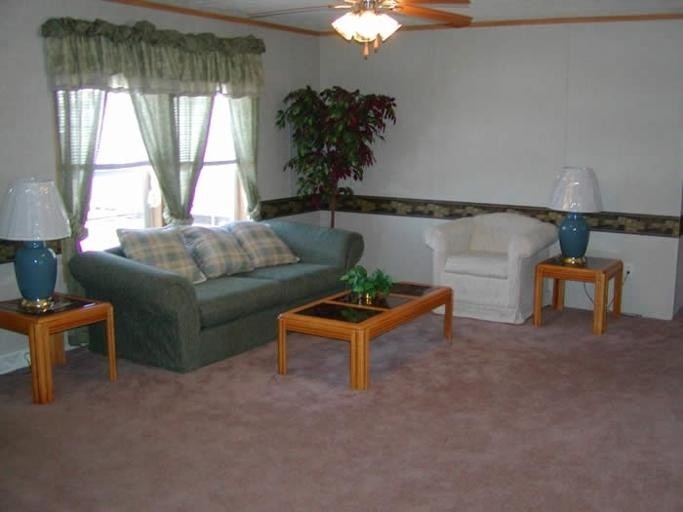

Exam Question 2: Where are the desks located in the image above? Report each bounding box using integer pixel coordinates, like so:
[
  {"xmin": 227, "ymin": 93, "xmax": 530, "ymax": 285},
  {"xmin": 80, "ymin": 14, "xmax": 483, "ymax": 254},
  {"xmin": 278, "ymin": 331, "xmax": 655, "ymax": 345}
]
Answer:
[
  {"xmin": 0, "ymin": 288, "xmax": 117, "ymax": 407},
  {"xmin": 529, "ymin": 255, "xmax": 625, "ymax": 338}
]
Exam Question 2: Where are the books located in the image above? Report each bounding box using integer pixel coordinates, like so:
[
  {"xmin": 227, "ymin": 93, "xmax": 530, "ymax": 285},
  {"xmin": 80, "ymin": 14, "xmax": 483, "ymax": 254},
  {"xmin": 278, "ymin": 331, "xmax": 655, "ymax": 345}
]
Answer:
[{"xmin": 70, "ymin": 297, "xmax": 95, "ymax": 308}]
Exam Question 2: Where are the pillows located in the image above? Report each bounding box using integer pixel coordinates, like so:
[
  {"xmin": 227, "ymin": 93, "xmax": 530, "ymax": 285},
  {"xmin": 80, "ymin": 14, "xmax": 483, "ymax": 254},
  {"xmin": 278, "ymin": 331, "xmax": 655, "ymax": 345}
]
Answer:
[
  {"xmin": 173, "ymin": 223, "xmax": 255, "ymax": 279},
  {"xmin": 227, "ymin": 216, "xmax": 300, "ymax": 272},
  {"xmin": 116, "ymin": 222, "xmax": 208, "ymax": 289}
]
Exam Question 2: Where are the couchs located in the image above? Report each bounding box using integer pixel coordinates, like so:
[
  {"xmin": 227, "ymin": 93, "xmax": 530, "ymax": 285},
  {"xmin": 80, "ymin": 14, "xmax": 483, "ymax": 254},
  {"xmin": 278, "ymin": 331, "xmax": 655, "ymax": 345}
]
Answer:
[{"xmin": 69, "ymin": 218, "xmax": 365, "ymax": 379}]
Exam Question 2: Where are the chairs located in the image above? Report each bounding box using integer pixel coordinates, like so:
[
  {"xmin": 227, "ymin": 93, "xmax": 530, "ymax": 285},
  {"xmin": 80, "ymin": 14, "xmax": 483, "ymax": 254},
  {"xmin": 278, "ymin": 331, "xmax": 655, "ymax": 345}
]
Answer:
[{"xmin": 422, "ymin": 211, "xmax": 562, "ymax": 329}]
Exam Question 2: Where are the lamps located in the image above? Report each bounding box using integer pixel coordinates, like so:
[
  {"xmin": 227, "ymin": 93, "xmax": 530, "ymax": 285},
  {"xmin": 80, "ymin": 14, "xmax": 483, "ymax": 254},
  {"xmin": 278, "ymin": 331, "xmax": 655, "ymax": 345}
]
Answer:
[
  {"xmin": 549, "ymin": 162, "xmax": 603, "ymax": 268},
  {"xmin": 334, "ymin": 10, "xmax": 403, "ymax": 59},
  {"xmin": 1, "ymin": 176, "xmax": 77, "ymax": 312}
]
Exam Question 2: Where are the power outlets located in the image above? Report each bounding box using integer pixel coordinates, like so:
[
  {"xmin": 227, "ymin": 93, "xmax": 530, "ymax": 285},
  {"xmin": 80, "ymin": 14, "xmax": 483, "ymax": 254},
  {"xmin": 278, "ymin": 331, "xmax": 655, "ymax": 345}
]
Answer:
[{"xmin": 623, "ymin": 262, "xmax": 632, "ymax": 280}]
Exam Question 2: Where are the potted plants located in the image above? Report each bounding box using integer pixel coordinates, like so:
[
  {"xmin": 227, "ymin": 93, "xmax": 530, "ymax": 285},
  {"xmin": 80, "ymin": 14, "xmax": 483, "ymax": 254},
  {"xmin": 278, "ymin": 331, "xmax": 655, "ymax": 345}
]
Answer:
[{"xmin": 340, "ymin": 265, "xmax": 391, "ymax": 305}]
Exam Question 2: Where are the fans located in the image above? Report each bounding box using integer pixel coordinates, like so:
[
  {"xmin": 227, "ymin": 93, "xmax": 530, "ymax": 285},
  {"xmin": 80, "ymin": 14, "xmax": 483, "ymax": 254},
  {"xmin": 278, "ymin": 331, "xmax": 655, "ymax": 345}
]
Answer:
[{"xmin": 246, "ymin": 0, "xmax": 472, "ymax": 38}]
